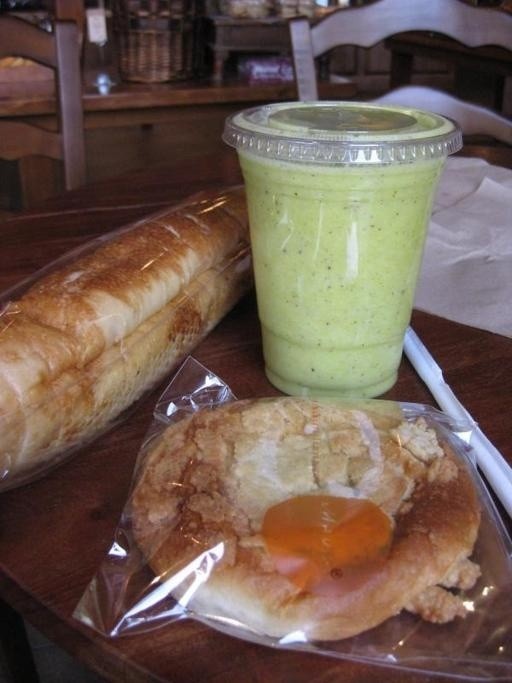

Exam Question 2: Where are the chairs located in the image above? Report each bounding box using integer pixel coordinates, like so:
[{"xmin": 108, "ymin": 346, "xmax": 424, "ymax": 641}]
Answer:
[
  {"xmin": 287, "ymin": 0, "xmax": 511, "ymax": 154},
  {"xmin": 1, "ymin": 11, "xmax": 88, "ymax": 210}
]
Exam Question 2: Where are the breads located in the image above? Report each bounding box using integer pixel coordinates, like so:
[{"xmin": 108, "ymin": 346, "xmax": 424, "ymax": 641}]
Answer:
[
  {"xmin": 1, "ymin": 186, "xmax": 254, "ymax": 487},
  {"xmin": 132, "ymin": 395, "xmax": 479, "ymax": 641}
]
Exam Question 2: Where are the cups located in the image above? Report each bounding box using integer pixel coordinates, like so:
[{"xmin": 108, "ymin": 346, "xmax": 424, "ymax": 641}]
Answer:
[{"xmin": 220, "ymin": 101, "xmax": 464, "ymax": 402}]
